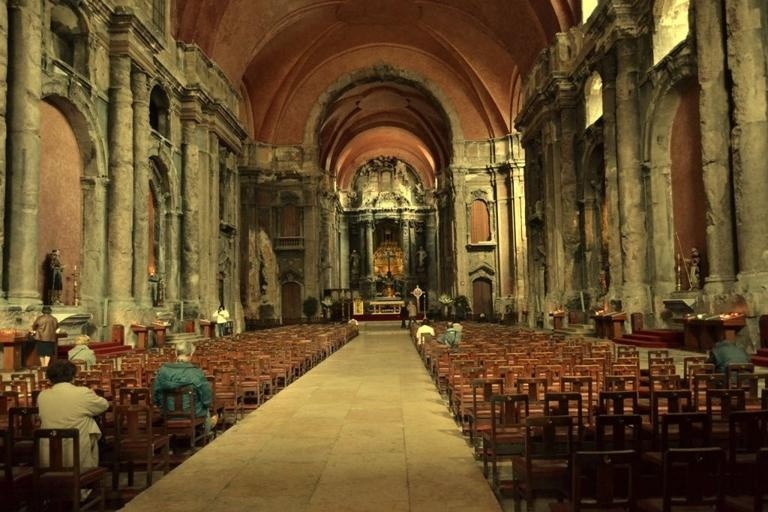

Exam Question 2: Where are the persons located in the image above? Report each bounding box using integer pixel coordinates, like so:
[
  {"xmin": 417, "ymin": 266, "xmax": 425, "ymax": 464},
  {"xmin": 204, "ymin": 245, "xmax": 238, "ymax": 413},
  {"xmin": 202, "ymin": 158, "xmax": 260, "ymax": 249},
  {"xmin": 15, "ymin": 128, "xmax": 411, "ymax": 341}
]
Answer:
[
  {"xmin": 36, "ymin": 359, "xmax": 109, "ymax": 501},
  {"xmin": 212, "ymin": 305, "xmax": 229, "ymax": 337},
  {"xmin": 685, "ymin": 248, "xmax": 701, "ymax": 292},
  {"xmin": 67, "ymin": 334, "xmax": 96, "ymax": 371},
  {"xmin": 349, "ymin": 250, "xmax": 361, "ymax": 273},
  {"xmin": 706, "ymin": 338, "xmax": 751, "ymax": 387},
  {"xmin": 400, "ymin": 304, "xmax": 463, "ymax": 352},
  {"xmin": 32, "ymin": 305, "xmax": 59, "ymax": 368},
  {"xmin": 42, "ymin": 248, "xmax": 64, "ymax": 305},
  {"xmin": 416, "ymin": 245, "xmax": 427, "ymax": 268},
  {"xmin": 150, "ymin": 341, "xmax": 218, "ymax": 435}
]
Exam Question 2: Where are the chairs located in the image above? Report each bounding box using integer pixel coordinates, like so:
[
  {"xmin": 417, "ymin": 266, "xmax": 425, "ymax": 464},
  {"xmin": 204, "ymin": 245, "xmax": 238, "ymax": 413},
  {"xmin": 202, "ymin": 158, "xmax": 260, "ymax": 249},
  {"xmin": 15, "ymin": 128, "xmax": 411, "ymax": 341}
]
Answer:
[
  {"xmin": 410, "ymin": 319, "xmax": 768, "ymax": 512},
  {"xmin": 0, "ymin": 323, "xmax": 359, "ymax": 512}
]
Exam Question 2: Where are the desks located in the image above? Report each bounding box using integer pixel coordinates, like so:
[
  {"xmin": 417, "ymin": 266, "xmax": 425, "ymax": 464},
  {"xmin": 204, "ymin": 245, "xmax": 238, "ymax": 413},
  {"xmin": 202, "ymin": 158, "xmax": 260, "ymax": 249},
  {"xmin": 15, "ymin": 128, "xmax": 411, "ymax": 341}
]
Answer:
[{"xmin": 369, "ymin": 300, "xmax": 406, "ymax": 315}]
